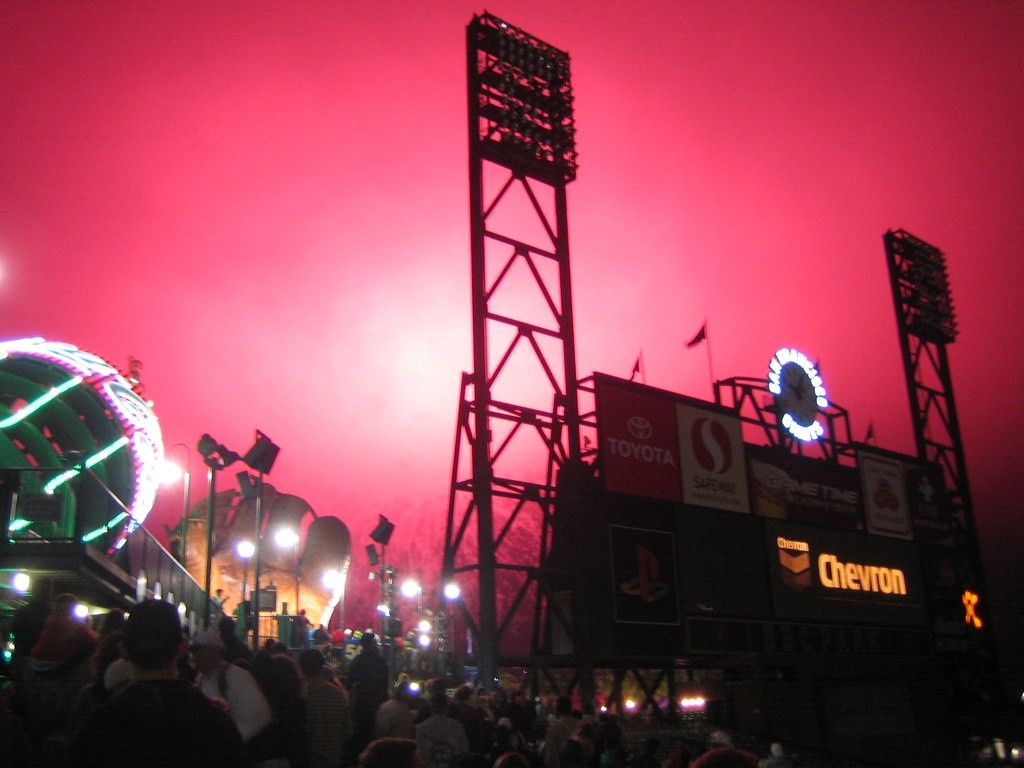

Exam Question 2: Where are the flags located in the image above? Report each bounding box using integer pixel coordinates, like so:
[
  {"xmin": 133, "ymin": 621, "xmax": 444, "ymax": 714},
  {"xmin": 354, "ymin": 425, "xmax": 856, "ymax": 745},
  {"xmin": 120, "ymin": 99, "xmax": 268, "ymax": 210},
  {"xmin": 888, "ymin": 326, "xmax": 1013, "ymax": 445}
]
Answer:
[
  {"xmin": 683, "ymin": 322, "xmax": 706, "ymax": 350},
  {"xmin": 628, "ymin": 353, "xmax": 642, "ymax": 381}
]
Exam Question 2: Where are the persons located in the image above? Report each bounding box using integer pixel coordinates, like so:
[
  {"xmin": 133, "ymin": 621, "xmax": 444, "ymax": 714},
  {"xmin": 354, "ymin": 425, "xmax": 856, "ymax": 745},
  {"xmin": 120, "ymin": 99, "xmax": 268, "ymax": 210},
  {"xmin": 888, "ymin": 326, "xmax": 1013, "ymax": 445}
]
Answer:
[
  {"xmin": 213, "ymin": 588, "xmax": 230, "ymax": 609},
  {"xmin": 295, "ymin": 609, "xmax": 315, "ymax": 647},
  {"xmin": 31, "ymin": 594, "xmax": 96, "ymax": 698},
  {"xmin": 11, "ymin": 577, "xmax": 51, "ymax": 655},
  {"xmin": 185, "ymin": 629, "xmax": 272, "ymax": 745},
  {"xmin": 64, "ymin": 597, "xmax": 242, "ymax": 768},
  {"xmin": 253, "ymin": 629, "xmax": 621, "ymax": 768},
  {"xmin": 9, "ymin": 578, "xmax": 303, "ymax": 768},
  {"xmin": 596, "ymin": 713, "xmax": 793, "ymax": 768}
]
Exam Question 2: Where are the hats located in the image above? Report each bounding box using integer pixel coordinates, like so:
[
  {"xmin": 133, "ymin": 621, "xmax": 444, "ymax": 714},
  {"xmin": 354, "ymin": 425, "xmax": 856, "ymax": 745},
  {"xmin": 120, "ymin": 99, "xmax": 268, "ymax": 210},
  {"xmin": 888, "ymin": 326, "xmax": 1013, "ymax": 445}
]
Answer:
[
  {"xmin": 428, "ymin": 690, "xmax": 447, "ymax": 710},
  {"xmin": 187, "ymin": 629, "xmax": 227, "ymax": 652},
  {"xmin": 103, "ymin": 660, "xmax": 134, "ymax": 691},
  {"xmin": 495, "ymin": 717, "xmax": 512, "ymax": 730}
]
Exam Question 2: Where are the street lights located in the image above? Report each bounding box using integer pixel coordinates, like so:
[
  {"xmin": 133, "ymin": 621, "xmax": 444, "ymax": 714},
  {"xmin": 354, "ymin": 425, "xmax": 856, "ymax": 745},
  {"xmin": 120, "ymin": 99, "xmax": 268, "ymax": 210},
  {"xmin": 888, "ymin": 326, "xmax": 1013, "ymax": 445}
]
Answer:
[
  {"xmin": 442, "ymin": 584, "xmax": 460, "ymax": 654},
  {"xmin": 155, "ymin": 442, "xmax": 194, "ymax": 604},
  {"xmin": 400, "ymin": 580, "xmax": 424, "ymax": 617},
  {"xmin": 274, "ymin": 528, "xmax": 300, "ymax": 614},
  {"xmin": 236, "ymin": 538, "xmax": 255, "ymax": 603}
]
